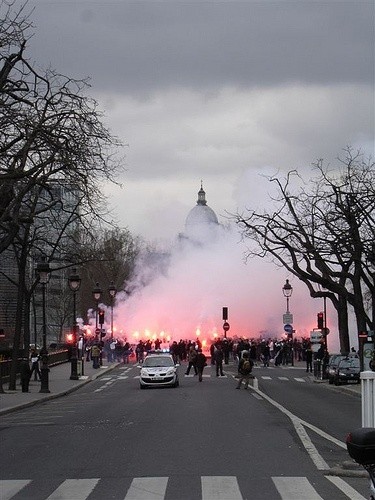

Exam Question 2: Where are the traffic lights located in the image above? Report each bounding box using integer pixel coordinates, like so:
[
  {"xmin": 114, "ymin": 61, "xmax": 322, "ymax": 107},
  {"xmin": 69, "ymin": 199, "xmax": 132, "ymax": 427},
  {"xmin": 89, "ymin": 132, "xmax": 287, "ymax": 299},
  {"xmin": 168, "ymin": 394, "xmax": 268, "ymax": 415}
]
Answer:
[
  {"xmin": 318, "ymin": 312, "xmax": 323, "ymax": 329},
  {"xmin": 223, "ymin": 307, "xmax": 228, "ymax": 320},
  {"xmin": 99, "ymin": 311, "xmax": 105, "ymax": 324}
]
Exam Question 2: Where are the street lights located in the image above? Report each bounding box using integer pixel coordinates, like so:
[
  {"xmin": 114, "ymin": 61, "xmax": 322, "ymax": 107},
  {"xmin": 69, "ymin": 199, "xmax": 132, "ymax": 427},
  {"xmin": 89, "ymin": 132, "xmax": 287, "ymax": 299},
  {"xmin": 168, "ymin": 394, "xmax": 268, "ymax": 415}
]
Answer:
[
  {"xmin": 281, "ymin": 279, "xmax": 293, "ymax": 314},
  {"xmin": 34, "ymin": 255, "xmax": 53, "ymax": 393},
  {"xmin": 108, "ymin": 281, "xmax": 117, "ymax": 338},
  {"xmin": 91, "ymin": 281, "xmax": 102, "ymax": 338},
  {"xmin": 68, "ymin": 267, "xmax": 83, "ymax": 380}
]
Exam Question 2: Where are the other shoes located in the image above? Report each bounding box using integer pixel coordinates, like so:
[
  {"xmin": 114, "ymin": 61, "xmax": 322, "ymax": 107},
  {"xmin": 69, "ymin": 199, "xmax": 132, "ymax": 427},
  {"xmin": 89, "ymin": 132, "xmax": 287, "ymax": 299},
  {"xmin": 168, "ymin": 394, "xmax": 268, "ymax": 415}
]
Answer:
[
  {"xmin": 216, "ymin": 375, "xmax": 220, "ymax": 376},
  {"xmin": 236, "ymin": 387, "xmax": 240, "ymax": 389},
  {"xmin": 221, "ymin": 374, "xmax": 225, "ymax": 376},
  {"xmin": 185, "ymin": 373, "xmax": 189, "ymax": 375},
  {"xmin": 245, "ymin": 387, "xmax": 247, "ymax": 389}
]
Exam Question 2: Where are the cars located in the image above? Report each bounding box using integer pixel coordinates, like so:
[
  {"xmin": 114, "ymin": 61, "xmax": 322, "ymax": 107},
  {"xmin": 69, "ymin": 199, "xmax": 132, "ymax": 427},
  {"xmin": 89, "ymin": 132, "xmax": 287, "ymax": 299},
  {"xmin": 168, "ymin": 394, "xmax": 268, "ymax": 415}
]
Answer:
[
  {"xmin": 137, "ymin": 350, "xmax": 181, "ymax": 388},
  {"xmin": 325, "ymin": 354, "xmax": 360, "ymax": 386}
]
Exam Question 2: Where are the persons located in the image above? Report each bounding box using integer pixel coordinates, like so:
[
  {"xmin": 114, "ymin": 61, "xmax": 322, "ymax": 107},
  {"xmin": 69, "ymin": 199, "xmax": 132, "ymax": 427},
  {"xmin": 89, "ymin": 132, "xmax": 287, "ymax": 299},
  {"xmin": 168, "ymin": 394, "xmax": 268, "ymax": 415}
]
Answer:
[{"xmin": 29, "ymin": 334, "xmax": 356, "ymax": 389}]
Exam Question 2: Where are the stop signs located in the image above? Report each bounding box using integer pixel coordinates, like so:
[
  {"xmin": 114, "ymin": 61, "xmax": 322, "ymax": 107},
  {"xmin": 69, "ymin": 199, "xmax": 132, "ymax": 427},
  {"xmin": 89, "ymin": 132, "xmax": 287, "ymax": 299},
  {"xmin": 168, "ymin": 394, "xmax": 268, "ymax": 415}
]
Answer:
[
  {"xmin": 95, "ymin": 329, "xmax": 101, "ymax": 335},
  {"xmin": 223, "ymin": 322, "xmax": 230, "ymax": 331},
  {"xmin": 0, "ymin": 333, "xmax": 7, "ymax": 340}
]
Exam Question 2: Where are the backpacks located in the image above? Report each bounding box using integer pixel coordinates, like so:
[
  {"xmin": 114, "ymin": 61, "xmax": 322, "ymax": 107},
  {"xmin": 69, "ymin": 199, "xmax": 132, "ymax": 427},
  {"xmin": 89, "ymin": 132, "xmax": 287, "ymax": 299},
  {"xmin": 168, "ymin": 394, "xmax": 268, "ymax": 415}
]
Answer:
[{"xmin": 243, "ymin": 358, "xmax": 251, "ymax": 369}]
